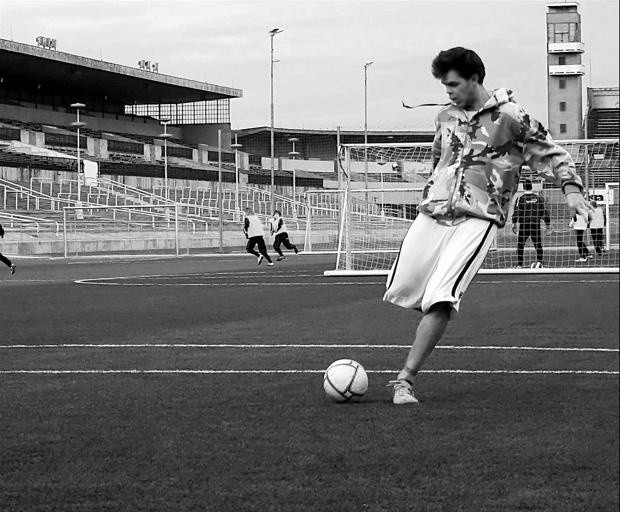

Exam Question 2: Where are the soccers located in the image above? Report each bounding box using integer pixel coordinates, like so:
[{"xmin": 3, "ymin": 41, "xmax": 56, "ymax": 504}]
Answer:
[{"xmin": 322, "ymin": 359, "xmax": 368, "ymax": 402}]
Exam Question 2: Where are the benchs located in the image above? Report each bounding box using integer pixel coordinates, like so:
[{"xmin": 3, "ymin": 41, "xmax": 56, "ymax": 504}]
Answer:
[{"xmin": 0, "ymin": 114, "xmax": 415, "ymax": 233}]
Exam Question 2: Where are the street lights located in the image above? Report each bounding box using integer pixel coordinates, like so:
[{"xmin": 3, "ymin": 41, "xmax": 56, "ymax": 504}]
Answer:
[
  {"xmin": 363, "ymin": 60, "xmax": 374, "ymax": 215},
  {"xmin": 288, "ymin": 138, "xmax": 298, "ymax": 222},
  {"xmin": 231, "ymin": 131, "xmax": 243, "ymax": 221},
  {"xmin": 70, "ymin": 102, "xmax": 87, "ymax": 207},
  {"xmin": 269, "ymin": 28, "xmax": 284, "ymax": 215},
  {"xmin": 156, "ymin": 118, "xmax": 171, "ymax": 226}
]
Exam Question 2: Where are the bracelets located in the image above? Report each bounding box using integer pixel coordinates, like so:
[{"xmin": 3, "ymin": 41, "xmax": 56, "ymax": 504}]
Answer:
[{"xmin": 564, "ymin": 191, "xmax": 582, "ymax": 196}]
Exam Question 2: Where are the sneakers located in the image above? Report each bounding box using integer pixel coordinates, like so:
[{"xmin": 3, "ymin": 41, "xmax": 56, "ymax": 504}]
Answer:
[
  {"xmin": 574, "ymin": 250, "xmax": 608, "ymax": 263},
  {"xmin": 11, "ymin": 264, "xmax": 16, "ymax": 275},
  {"xmin": 392, "ymin": 379, "xmax": 420, "ymax": 406},
  {"xmin": 257, "ymin": 246, "xmax": 298, "ymax": 266},
  {"xmin": 515, "ymin": 265, "xmax": 524, "ymax": 269}
]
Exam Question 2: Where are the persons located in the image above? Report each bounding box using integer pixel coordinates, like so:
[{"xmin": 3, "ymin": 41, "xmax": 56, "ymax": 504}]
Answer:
[
  {"xmin": 381, "ymin": 45, "xmax": 597, "ymax": 408},
  {"xmin": 240, "ymin": 207, "xmax": 275, "ymax": 266},
  {"xmin": 587, "ymin": 200, "xmax": 608, "ymax": 256},
  {"xmin": 0, "ymin": 223, "xmax": 18, "ymax": 277},
  {"xmin": 568, "ymin": 208, "xmax": 594, "ymax": 264},
  {"xmin": 269, "ymin": 209, "xmax": 300, "ymax": 262},
  {"xmin": 511, "ymin": 180, "xmax": 553, "ymax": 269}
]
